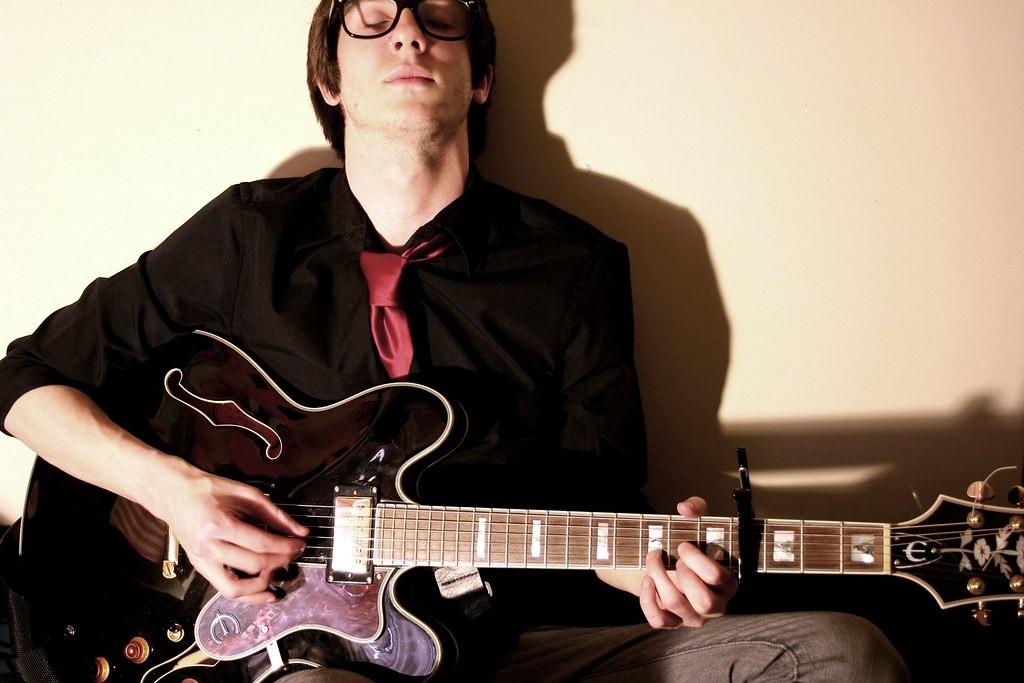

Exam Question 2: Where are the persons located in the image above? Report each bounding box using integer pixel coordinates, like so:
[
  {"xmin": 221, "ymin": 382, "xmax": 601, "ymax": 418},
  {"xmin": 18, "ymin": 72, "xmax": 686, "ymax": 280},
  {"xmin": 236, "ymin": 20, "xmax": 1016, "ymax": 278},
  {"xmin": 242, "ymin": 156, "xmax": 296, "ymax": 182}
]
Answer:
[{"xmin": 0, "ymin": 0, "xmax": 912, "ymax": 683}]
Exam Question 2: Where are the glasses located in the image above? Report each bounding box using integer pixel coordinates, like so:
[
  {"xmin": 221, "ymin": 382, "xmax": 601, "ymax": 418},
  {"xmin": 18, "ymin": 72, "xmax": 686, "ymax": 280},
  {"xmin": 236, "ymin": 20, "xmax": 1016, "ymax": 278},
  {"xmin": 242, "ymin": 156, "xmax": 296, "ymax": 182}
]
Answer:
[{"xmin": 326, "ymin": 0, "xmax": 486, "ymax": 62}]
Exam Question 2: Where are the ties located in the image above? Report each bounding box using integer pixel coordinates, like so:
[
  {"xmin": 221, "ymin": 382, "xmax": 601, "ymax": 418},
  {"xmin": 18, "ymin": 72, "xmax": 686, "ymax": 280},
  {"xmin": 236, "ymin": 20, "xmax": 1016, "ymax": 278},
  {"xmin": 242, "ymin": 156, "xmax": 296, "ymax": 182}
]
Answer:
[{"xmin": 359, "ymin": 231, "xmax": 444, "ymax": 381}]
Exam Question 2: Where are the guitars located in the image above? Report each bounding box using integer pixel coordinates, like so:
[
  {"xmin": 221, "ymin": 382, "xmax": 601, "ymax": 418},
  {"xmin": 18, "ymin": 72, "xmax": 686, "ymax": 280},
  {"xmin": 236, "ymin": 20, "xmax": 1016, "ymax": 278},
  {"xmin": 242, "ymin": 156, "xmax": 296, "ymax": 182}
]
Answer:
[{"xmin": 18, "ymin": 330, "xmax": 1023, "ymax": 683}]
